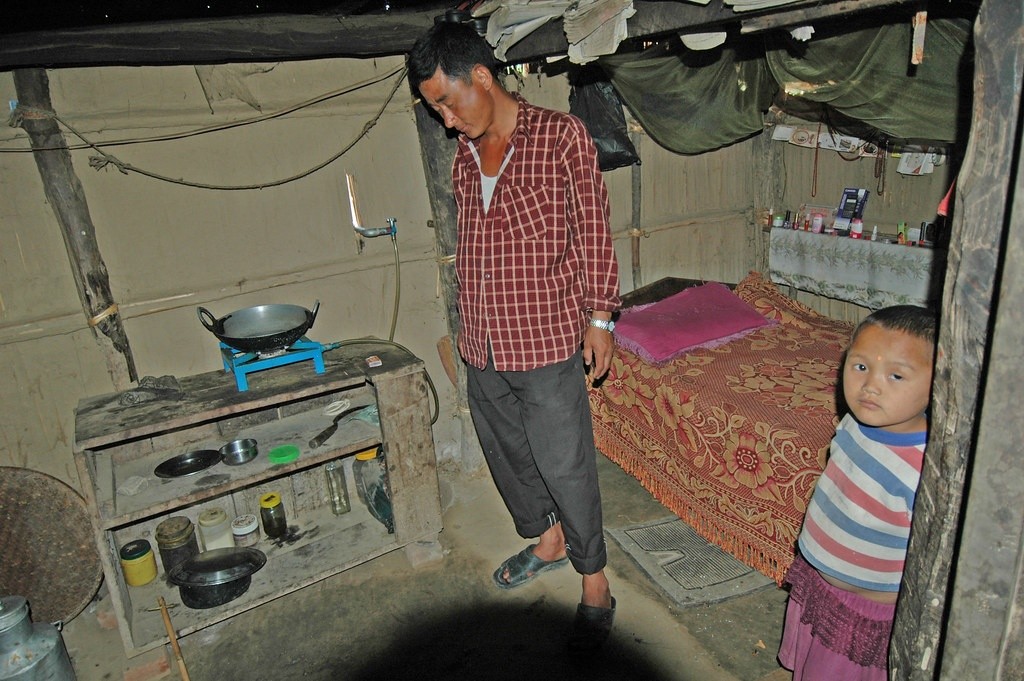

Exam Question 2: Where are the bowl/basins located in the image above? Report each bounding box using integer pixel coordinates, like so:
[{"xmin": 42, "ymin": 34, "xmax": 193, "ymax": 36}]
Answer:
[{"xmin": 219, "ymin": 439, "xmax": 258, "ymax": 466}]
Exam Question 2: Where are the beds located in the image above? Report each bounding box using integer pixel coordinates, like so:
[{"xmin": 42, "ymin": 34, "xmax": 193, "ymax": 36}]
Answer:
[{"xmin": 585, "ymin": 271, "xmax": 860, "ymax": 591}]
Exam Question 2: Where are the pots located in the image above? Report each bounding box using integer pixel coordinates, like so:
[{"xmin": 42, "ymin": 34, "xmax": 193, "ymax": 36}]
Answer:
[
  {"xmin": 197, "ymin": 300, "xmax": 320, "ymax": 353},
  {"xmin": 179, "ymin": 577, "xmax": 252, "ymax": 609}
]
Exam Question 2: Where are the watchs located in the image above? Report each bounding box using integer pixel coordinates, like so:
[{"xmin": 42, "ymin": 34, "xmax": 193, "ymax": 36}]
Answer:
[{"xmin": 590, "ymin": 319, "xmax": 615, "ymax": 332}]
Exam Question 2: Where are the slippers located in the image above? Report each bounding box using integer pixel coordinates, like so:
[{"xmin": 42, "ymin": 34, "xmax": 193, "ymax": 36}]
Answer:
[
  {"xmin": 493, "ymin": 544, "xmax": 570, "ymax": 590},
  {"xmin": 567, "ymin": 595, "xmax": 617, "ymax": 658}
]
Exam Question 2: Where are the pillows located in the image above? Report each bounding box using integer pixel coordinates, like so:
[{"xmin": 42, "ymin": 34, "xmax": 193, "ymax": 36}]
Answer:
[{"xmin": 611, "ymin": 281, "xmax": 779, "ymax": 369}]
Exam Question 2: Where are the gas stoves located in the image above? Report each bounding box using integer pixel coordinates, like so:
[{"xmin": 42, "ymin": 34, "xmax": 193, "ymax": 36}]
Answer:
[{"xmin": 219, "ymin": 336, "xmax": 325, "ymax": 392}]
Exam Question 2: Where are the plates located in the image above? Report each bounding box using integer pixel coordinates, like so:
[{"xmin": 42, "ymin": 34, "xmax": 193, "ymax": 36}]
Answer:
[
  {"xmin": 168, "ymin": 547, "xmax": 267, "ymax": 587},
  {"xmin": 154, "ymin": 449, "xmax": 223, "ymax": 479}
]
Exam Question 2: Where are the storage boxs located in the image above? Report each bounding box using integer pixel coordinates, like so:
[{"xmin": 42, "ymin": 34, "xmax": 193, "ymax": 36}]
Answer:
[
  {"xmin": 897, "ymin": 222, "xmax": 907, "ymax": 245},
  {"xmin": 832, "ymin": 187, "xmax": 869, "ymax": 231}
]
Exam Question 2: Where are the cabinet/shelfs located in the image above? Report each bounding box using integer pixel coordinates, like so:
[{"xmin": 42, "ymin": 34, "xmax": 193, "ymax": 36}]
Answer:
[{"xmin": 72, "ymin": 336, "xmax": 445, "ymax": 659}]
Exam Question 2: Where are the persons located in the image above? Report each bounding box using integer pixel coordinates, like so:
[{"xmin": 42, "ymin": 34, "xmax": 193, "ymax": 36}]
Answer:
[
  {"xmin": 777, "ymin": 304, "xmax": 940, "ymax": 681},
  {"xmin": 408, "ymin": 20, "xmax": 623, "ymax": 663}
]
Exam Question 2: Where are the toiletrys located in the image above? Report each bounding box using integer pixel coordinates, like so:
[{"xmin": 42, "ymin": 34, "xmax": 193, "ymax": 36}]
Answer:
[
  {"xmin": 763, "ymin": 208, "xmax": 891, "ymax": 244},
  {"xmin": 919, "ymin": 222, "xmax": 925, "ymax": 246}
]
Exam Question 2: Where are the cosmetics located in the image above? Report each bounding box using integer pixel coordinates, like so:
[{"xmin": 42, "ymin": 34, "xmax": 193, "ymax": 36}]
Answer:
[{"xmin": 771, "ymin": 208, "xmax": 916, "ymax": 246}]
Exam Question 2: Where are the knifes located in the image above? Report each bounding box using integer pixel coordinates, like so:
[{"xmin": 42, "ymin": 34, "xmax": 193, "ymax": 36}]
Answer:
[{"xmin": 308, "ymin": 404, "xmax": 370, "ymax": 449}]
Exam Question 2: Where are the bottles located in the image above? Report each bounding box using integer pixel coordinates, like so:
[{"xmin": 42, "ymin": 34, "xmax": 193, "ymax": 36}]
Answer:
[
  {"xmin": 260, "ymin": 492, "xmax": 287, "ymax": 537},
  {"xmin": 232, "ymin": 514, "xmax": 261, "ymax": 547},
  {"xmin": 325, "ymin": 459, "xmax": 351, "ymax": 518}
]
